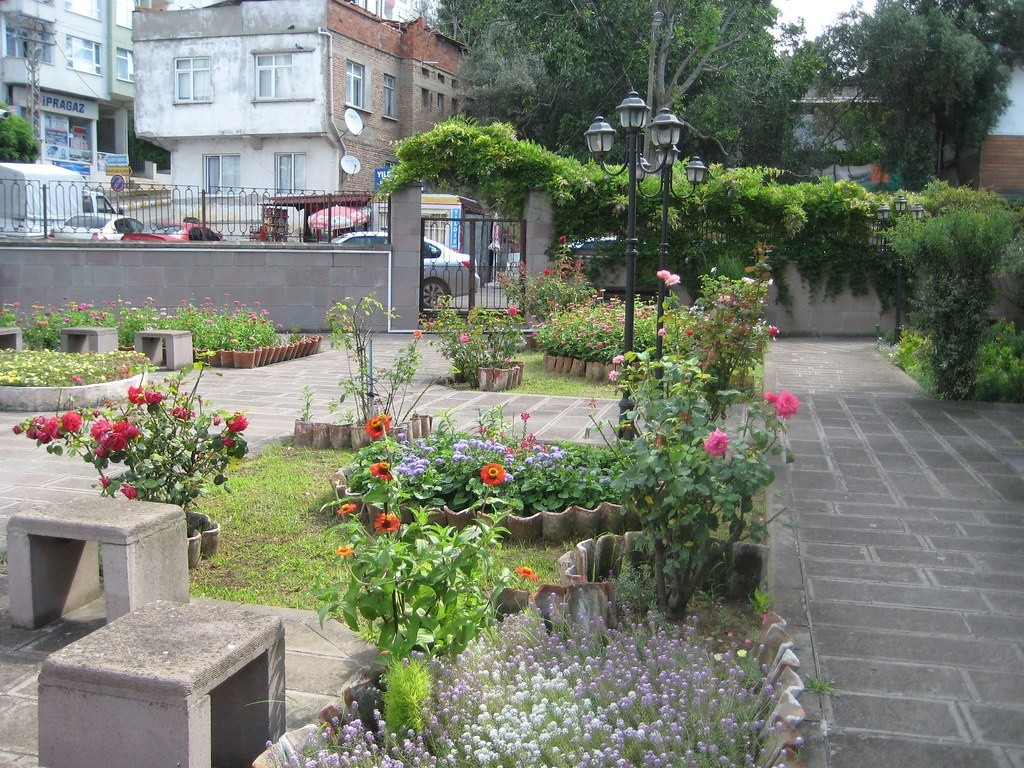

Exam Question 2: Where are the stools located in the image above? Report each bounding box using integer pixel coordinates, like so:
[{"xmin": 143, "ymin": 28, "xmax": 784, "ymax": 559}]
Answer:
[
  {"xmin": 135, "ymin": 329, "xmax": 193, "ymax": 371},
  {"xmin": 61, "ymin": 327, "xmax": 118, "ymax": 354},
  {"xmin": 5, "ymin": 495, "xmax": 191, "ymax": 630},
  {"xmin": 37, "ymin": 600, "xmax": 288, "ymax": 768},
  {"xmin": 0, "ymin": 326, "xmax": 23, "ymax": 350}
]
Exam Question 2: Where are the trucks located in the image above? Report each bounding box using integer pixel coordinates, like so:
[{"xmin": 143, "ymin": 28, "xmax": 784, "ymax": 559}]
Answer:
[
  {"xmin": 0, "ymin": 162, "xmax": 125, "ymax": 237},
  {"xmin": 369, "ymin": 193, "xmax": 504, "ymax": 287}
]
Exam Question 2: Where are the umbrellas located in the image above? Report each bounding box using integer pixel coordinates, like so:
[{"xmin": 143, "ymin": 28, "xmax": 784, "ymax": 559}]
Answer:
[
  {"xmin": 308, "ymin": 204, "xmax": 370, "ymax": 237},
  {"xmin": 487, "ymin": 212, "xmax": 502, "ymax": 284}
]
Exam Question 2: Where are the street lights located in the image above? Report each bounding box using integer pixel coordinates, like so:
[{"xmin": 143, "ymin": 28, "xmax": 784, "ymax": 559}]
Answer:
[
  {"xmin": 583, "ymin": 90, "xmax": 687, "ymax": 447},
  {"xmin": 869, "ymin": 194, "xmax": 926, "ymax": 351},
  {"xmin": 624, "ymin": 150, "xmax": 708, "ymax": 407}
]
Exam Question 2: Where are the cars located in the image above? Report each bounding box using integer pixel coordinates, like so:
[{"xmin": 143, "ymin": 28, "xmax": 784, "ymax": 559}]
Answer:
[
  {"xmin": 324, "ymin": 231, "xmax": 483, "ymax": 308},
  {"xmin": 48, "ymin": 212, "xmax": 145, "ymax": 243},
  {"xmin": 122, "ymin": 220, "xmax": 224, "ymax": 242}
]
